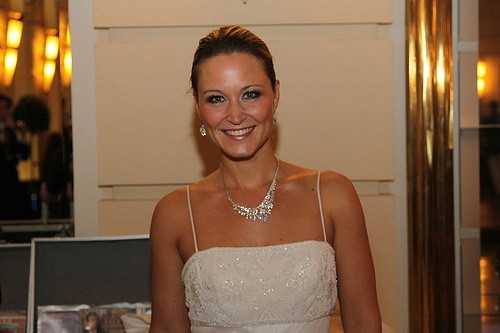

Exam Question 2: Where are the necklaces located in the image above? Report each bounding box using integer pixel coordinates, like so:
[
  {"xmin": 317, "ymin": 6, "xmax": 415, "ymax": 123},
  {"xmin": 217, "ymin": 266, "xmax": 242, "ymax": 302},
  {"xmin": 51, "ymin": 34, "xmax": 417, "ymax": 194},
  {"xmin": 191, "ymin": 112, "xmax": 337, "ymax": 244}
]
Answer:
[{"xmin": 219, "ymin": 153, "xmax": 279, "ymax": 223}]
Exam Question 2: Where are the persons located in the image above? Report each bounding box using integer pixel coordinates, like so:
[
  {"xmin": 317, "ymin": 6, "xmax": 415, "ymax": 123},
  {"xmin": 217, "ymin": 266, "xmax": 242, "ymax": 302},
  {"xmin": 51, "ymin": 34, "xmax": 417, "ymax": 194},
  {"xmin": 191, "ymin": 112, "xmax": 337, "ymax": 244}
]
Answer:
[
  {"xmin": 1, "ymin": 93, "xmax": 31, "ymax": 221},
  {"xmin": 150, "ymin": 26, "xmax": 383, "ymax": 333}
]
